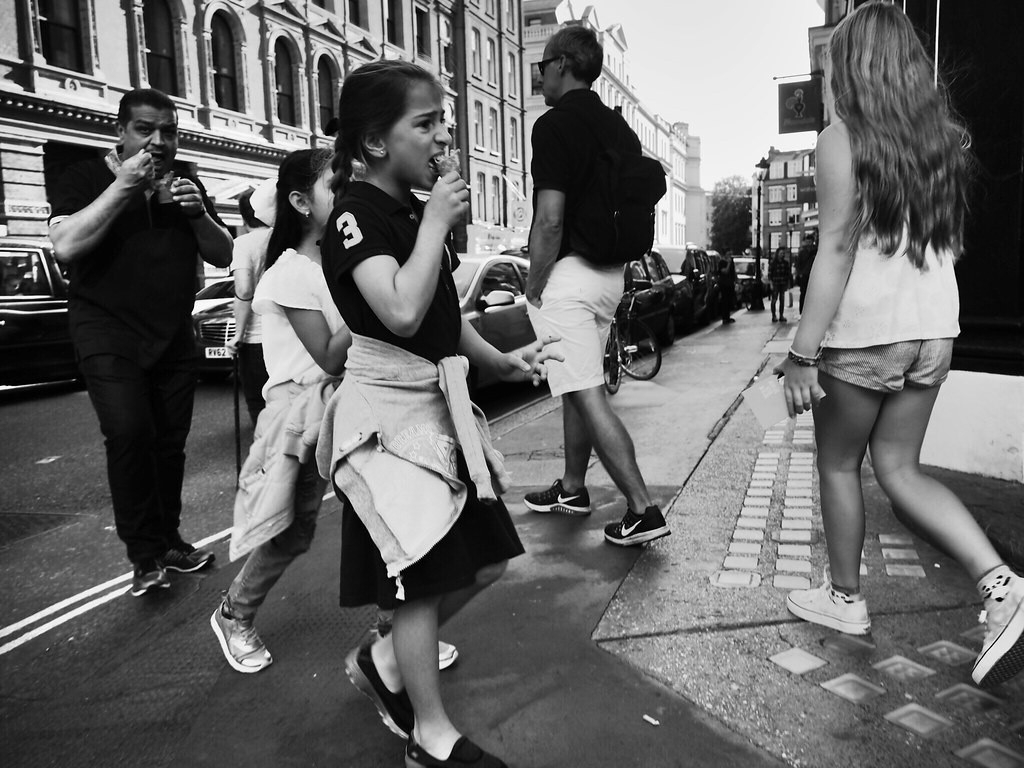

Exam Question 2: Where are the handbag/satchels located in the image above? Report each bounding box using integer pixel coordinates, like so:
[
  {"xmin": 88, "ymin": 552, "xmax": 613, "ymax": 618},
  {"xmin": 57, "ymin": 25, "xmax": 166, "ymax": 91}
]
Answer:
[{"xmin": 783, "ymin": 280, "xmax": 794, "ymax": 308}]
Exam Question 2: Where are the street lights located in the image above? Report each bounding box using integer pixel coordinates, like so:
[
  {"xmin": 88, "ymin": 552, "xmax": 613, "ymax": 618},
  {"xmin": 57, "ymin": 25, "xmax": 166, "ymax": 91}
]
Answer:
[{"xmin": 750, "ymin": 158, "xmax": 770, "ymax": 311}]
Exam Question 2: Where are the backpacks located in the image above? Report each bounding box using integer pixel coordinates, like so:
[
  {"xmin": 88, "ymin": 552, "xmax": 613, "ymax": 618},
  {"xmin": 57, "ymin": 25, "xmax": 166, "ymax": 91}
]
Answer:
[{"xmin": 557, "ymin": 101, "xmax": 660, "ymax": 268}]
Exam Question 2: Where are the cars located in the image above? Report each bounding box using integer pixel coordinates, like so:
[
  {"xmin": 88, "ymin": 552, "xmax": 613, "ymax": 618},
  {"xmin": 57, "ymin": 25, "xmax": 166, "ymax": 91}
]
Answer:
[
  {"xmin": 617, "ymin": 250, "xmax": 676, "ymax": 363},
  {"xmin": 706, "ymin": 249, "xmax": 769, "ymax": 323},
  {"xmin": 452, "ymin": 251, "xmax": 536, "ymax": 417},
  {"xmin": 0, "ymin": 237, "xmax": 85, "ymax": 393},
  {"xmin": 190, "ymin": 280, "xmax": 242, "ymax": 395}
]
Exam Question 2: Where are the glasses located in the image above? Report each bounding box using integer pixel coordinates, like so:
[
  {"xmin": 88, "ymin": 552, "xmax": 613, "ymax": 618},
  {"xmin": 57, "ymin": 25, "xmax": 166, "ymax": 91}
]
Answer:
[{"xmin": 538, "ymin": 53, "xmax": 581, "ymax": 76}]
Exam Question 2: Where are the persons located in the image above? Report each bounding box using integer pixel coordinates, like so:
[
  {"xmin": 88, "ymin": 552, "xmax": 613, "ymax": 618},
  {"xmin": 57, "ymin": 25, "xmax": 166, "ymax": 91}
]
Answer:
[
  {"xmin": 795, "ymin": 234, "xmax": 819, "ymax": 318},
  {"xmin": 227, "ymin": 185, "xmax": 270, "ymax": 429},
  {"xmin": 212, "ymin": 147, "xmax": 460, "ymax": 675},
  {"xmin": 718, "ymin": 246, "xmax": 737, "ymax": 324},
  {"xmin": 47, "ymin": 89, "xmax": 235, "ymax": 598},
  {"xmin": 320, "ymin": 59, "xmax": 567, "ymax": 766},
  {"xmin": 522, "ymin": 25, "xmax": 671, "ymax": 545},
  {"xmin": 769, "ymin": 248, "xmax": 792, "ymax": 321},
  {"xmin": 783, "ymin": 1, "xmax": 1023, "ymax": 686}
]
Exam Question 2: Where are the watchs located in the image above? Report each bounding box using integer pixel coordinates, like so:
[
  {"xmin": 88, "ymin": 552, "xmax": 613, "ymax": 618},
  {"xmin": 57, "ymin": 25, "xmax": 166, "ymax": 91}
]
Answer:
[{"xmin": 789, "ymin": 347, "xmax": 825, "ymax": 367}]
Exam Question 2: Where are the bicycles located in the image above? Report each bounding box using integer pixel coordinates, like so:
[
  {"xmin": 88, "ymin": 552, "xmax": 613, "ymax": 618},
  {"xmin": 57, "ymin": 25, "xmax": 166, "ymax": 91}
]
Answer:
[{"xmin": 602, "ymin": 288, "xmax": 662, "ymax": 395}]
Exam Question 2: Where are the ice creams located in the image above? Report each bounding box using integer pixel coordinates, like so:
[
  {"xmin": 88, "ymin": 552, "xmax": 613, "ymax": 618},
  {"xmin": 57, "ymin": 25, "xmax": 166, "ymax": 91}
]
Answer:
[{"xmin": 432, "ymin": 147, "xmax": 461, "ymax": 177}]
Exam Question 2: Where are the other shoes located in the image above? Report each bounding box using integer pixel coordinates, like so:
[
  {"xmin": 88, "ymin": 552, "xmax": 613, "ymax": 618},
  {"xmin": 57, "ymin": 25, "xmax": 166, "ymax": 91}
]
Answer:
[
  {"xmin": 401, "ymin": 727, "xmax": 509, "ymax": 768},
  {"xmin": 778, "ymin": 317, "xmax": 788, "ymax": 322},
  {"xmin": 721, "ymin": 318, "xmax": 736, "ymax": 325},
  {"xmin": 771, "ymin": 317, "xmax": 779, "ymax": 322},
  {"xmin": 346, "ymin": 640, "xmax": 416, "ymax": 739}
]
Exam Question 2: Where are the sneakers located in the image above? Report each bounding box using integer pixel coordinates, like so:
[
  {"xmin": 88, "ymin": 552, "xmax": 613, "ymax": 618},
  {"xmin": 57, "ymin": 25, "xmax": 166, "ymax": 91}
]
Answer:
[
  {"xmin": 370, "ymin": 621, "xmax": 460, "ymax": 669},
  {"xmin": 523, "ymin": 478, "xmax": 593, "ymax": 515},
  {"xmin": 155, "ymin": 544, "xmax": 215, "ymax": 572},
  {"xmin": 603, "ymin": 500, "xmax": 671, "ymax": 546},
  {"xmin": 971, "ymin": 575, "xmax": 1024, "ymax": 689},
  {"xmin": 129, "ymin": 556, "xmax": 170, "ymax": 597},
  {"xmin": 785, "ymin": 563, "xmax": 873, "ymax": 637},
  {"xmin": 209, "ymin": 601, "xmax": 274, "ymax": 675}
]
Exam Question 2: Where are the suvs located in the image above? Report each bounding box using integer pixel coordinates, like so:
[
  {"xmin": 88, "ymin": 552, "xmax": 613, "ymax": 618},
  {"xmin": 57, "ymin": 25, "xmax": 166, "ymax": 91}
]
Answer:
[{"xmin": 653, "ymin": 243, "xmax": 710, "ymax": 340}]
